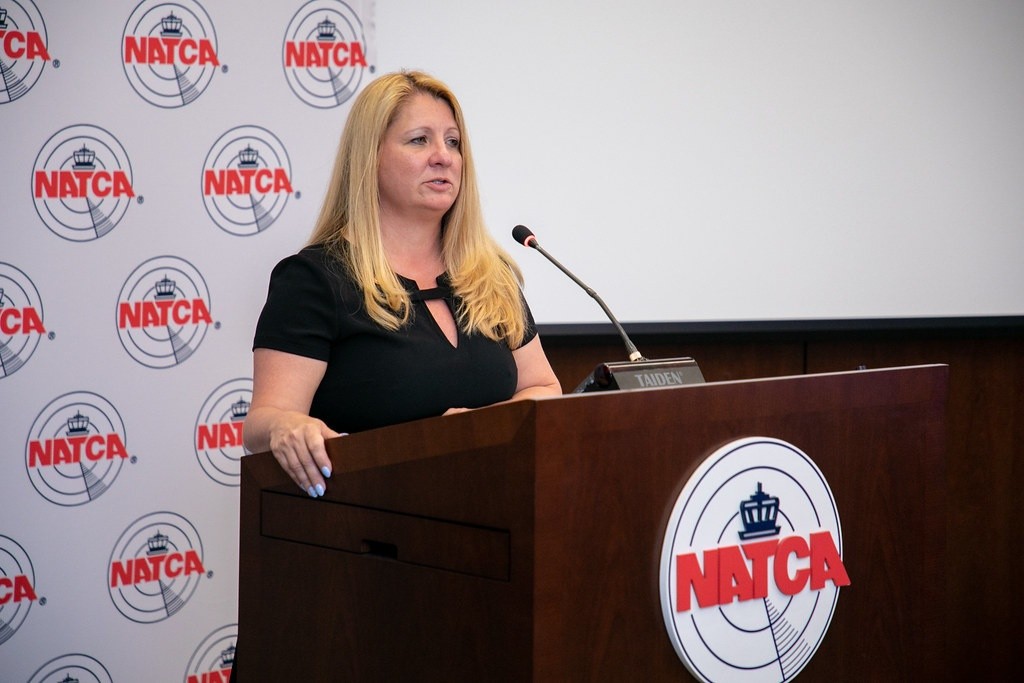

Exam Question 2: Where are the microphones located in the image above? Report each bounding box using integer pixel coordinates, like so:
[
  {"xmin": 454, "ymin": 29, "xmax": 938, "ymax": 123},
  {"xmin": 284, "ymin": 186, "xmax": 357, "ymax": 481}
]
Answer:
[{"xmin": 512, "ymin": 224, "xmax": 705, "ymax": 394}]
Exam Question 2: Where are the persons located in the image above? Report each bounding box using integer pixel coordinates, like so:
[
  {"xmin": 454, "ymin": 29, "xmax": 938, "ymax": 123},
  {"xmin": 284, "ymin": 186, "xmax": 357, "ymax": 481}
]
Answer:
[{"xmin": 242, "ymin": 72, "xmax": 562, "ymax": 497}]
